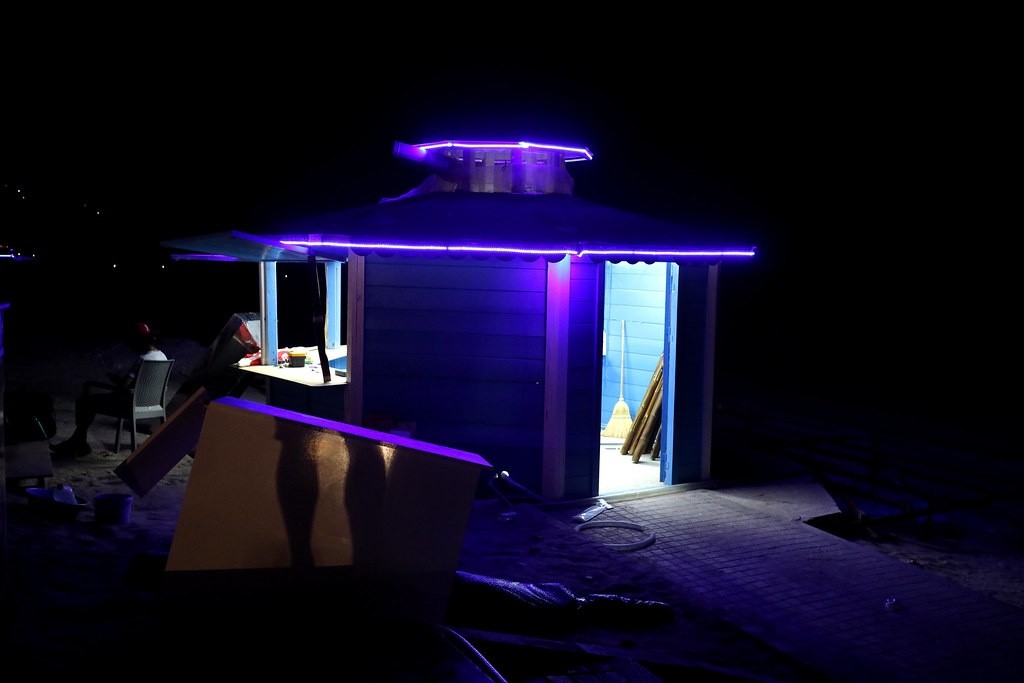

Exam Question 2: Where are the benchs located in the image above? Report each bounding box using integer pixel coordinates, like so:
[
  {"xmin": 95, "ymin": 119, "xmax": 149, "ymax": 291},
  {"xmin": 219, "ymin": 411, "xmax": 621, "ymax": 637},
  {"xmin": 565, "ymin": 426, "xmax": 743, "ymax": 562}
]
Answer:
[{"xmin": 3, "ymin": 438, "xmax": 55, "ymax": 490}]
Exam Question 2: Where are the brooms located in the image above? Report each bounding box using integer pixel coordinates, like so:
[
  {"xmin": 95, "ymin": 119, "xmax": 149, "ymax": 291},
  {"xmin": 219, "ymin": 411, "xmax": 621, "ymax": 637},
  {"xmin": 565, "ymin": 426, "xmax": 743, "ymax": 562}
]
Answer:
[{"xmin": 600, "ymin": 320, "xmax": 633, "ymax": 438}]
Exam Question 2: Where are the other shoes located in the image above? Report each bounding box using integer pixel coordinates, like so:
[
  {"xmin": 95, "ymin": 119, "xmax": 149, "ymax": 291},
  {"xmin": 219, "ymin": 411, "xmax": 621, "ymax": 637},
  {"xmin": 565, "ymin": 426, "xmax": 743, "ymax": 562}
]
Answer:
[{"xmin": 48, "ymin": 440, "xmax": 91, "ymax": 457}]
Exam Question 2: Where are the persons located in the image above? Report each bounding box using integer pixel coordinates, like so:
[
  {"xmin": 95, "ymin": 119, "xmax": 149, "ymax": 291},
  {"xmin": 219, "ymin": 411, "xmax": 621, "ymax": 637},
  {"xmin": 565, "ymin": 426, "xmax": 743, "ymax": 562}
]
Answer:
[{"xmin": 51, "ymin": 321, "xmax": 169, "ymax": 458}]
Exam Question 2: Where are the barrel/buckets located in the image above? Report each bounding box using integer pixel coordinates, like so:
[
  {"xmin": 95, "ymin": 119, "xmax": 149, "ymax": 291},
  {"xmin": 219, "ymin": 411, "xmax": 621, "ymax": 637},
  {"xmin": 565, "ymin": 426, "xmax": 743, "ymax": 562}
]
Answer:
[{"xmin": 94, "ymin": 494, "xmax": 133, "ymax": 523}]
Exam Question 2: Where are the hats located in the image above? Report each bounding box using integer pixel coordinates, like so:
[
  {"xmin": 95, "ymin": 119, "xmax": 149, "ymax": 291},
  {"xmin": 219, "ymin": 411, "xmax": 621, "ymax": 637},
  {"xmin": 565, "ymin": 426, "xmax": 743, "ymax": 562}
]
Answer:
[{"xmin": 120, "ymin": 323, "xmax": 151, "ymax": 345}]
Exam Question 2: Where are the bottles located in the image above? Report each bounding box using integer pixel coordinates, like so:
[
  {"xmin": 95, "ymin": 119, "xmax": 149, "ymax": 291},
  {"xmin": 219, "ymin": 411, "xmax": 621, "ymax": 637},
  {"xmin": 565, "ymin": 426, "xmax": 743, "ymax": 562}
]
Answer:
[{"xmin": 54, "ymin": 483, "xmax": 78, "ymax": 505}]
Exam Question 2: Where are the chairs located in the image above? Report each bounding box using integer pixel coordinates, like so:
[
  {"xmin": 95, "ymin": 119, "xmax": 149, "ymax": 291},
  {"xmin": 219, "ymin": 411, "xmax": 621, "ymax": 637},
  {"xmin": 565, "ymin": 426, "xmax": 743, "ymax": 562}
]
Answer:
[{"xmin": 86, "ymin": 358, "xmax": 176, "ymax": 454}]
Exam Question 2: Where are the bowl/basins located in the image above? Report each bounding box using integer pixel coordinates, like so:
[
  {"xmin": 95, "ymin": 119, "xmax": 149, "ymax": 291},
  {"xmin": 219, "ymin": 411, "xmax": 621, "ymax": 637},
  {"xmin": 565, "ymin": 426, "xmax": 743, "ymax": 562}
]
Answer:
[{"xmin": 26, "ymin": 488, "xmax": 90, "ymax": 520}]
